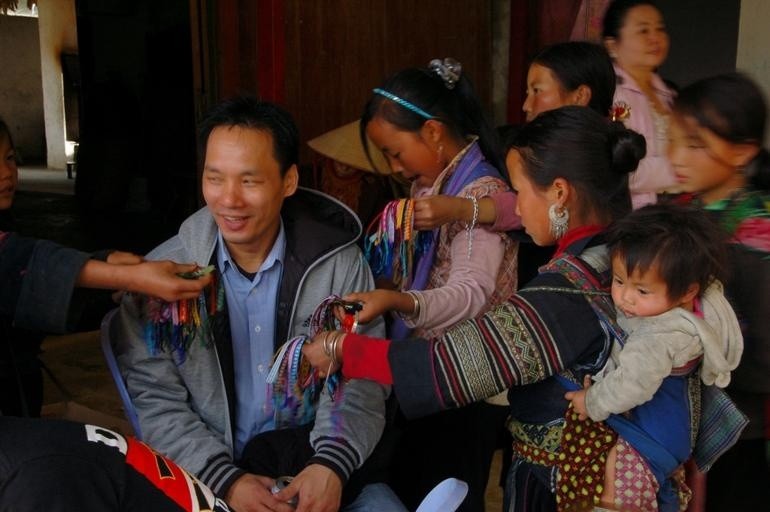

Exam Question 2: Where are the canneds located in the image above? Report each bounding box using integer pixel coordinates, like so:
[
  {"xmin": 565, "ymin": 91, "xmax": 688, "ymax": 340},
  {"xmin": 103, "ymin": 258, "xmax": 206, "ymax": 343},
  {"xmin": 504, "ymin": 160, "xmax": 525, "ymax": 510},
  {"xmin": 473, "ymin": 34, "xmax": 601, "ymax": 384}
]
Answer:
[{"xmin": 269, "ymin": 477, "xmax": 297, "ymax": 506}]
